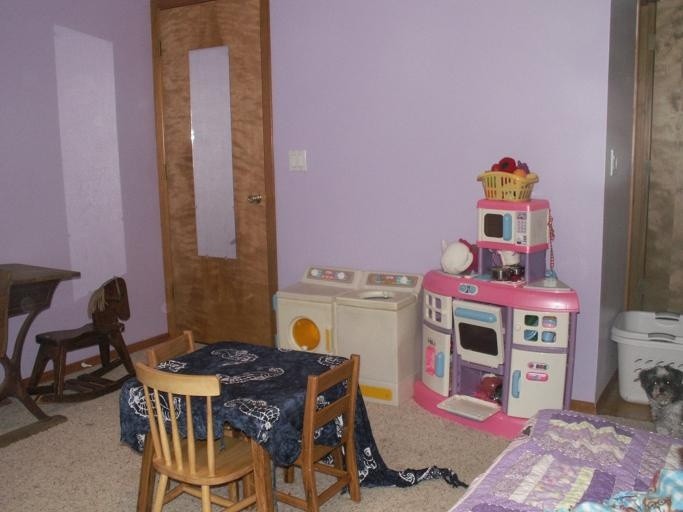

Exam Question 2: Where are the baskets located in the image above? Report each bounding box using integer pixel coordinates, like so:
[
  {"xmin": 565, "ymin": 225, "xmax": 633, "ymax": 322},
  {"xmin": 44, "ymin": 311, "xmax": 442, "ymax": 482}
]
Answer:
[{"xmin": 476, "ymin": 171, "xmax": 539, "ymax": 202}]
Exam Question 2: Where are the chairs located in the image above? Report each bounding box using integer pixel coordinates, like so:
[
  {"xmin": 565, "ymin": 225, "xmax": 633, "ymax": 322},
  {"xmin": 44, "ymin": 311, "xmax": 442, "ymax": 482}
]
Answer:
[{"xmin": 134, "ymin": 330, "xmax": 362, "ymax": 512}]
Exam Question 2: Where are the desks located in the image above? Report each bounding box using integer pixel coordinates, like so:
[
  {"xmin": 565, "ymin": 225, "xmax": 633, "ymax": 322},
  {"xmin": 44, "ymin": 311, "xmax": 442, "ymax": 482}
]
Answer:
[
  {"xmin": 118, "ymin": 340, "xmax": 351, "ymax": 512},
  {"xmin": 0, "ymin": 262, "xmax": 83, "ymax": 450}
]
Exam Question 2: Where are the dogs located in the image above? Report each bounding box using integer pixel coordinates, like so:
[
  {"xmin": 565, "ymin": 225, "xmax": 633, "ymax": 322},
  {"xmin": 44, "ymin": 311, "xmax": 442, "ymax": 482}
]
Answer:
[{"xmin": 638, "ymin": 365, "xmax": 682, "ymax": 439}]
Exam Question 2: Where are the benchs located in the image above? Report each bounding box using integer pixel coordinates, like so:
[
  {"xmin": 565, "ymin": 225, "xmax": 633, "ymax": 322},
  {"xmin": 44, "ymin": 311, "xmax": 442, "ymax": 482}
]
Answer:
[{"xmin": 442, "ymin": 404, "xmax": 683, "ymax": 512}]
